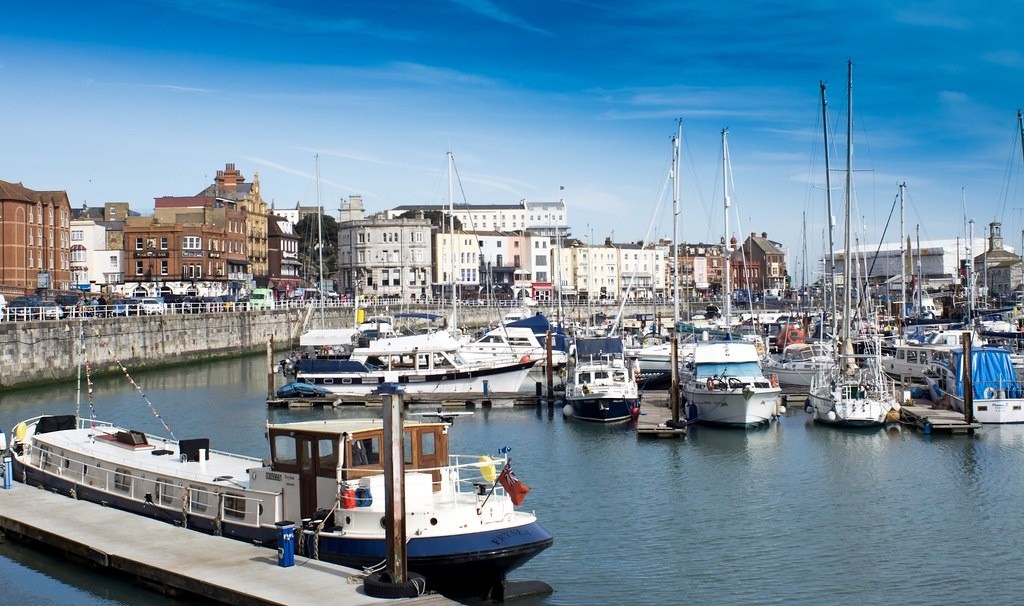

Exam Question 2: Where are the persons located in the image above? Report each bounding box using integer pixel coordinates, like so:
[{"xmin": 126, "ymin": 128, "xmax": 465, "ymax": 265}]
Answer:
[
  {"xmin": 78, "ymin": 295, "xmax": 106, "ymax": 318},
  {"xmin": 309, "ymin": 291, "xmax": 400, "ymax": 304}
]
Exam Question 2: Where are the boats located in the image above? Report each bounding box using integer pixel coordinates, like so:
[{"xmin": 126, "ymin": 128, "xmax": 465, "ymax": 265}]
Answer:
[{"xmin": 6, "ymin": 309, "xmax": 555, "ymax": 598}]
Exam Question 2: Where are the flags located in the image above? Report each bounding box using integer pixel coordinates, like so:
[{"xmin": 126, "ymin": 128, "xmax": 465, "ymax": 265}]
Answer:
[{"xmin": 498, "ymin": 463, "xmax": 531, "ymax": 505}]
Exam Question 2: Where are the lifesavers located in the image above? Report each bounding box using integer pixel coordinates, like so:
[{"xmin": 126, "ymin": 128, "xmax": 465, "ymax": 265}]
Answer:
[
  {"xmin": 984, "ymin": 387, "xmax": 998, "ymax": 400},
  {"xmin": 478, "ymin": 457, "xmax": 497, "ymax": 484},
  {"xmin": 17, "ymin": 423, "xmax": 27, "ymax": 438},
  {"xmin": 771, "ymin": 374, "xmax": 777, "ymax": 388},
  {"xmin": 706, "ymin": 377, "xmax": 715, "ymax": 391}
]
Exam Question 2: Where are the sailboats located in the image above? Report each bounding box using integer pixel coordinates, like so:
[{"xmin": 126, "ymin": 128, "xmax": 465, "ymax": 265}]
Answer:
[{"xmin": 274, "ymin": 57, "xmax": 1024, "ymax": 436}]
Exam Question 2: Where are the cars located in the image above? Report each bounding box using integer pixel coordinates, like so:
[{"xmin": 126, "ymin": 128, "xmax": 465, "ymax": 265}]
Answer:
[{"xmin": 3, "ymin": 292, "xmax": 249, "ymax": 321}]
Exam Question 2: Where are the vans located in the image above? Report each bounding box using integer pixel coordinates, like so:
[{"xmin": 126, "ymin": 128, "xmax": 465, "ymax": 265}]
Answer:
[{"xmin": 248, "ymin": 288, "xmax": 274, "ymax": 309}]
